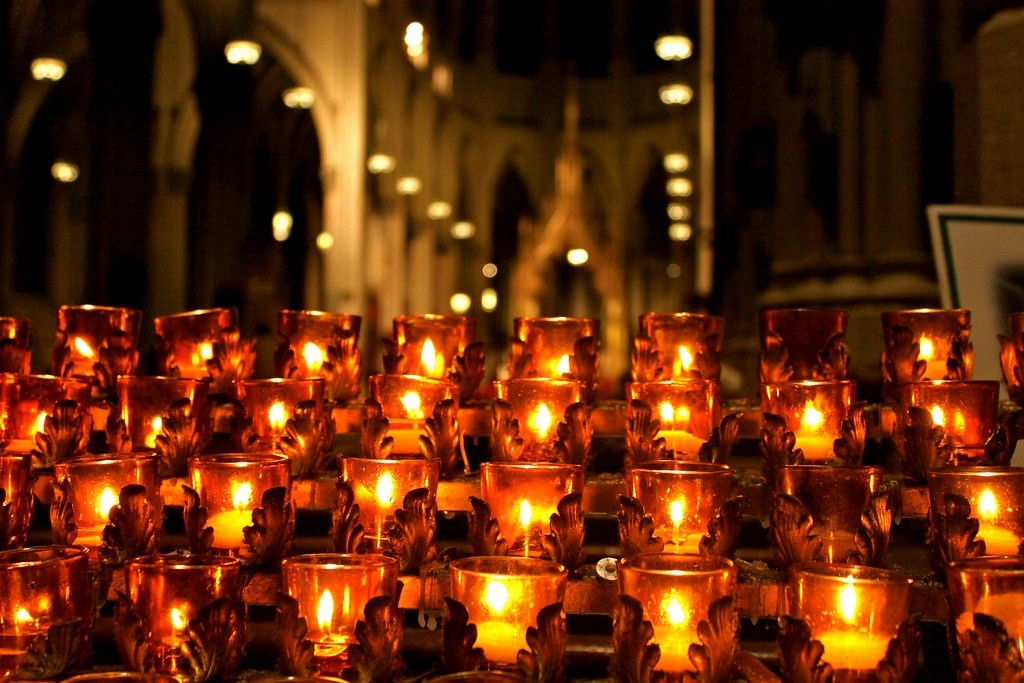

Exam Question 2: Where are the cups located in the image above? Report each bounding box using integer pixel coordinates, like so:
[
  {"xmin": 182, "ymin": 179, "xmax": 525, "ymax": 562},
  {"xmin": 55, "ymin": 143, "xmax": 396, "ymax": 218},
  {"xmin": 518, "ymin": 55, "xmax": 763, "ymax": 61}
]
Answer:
[{"xmin": 0, "ymin": 299, "xmax": 1024, "ymax": 683}]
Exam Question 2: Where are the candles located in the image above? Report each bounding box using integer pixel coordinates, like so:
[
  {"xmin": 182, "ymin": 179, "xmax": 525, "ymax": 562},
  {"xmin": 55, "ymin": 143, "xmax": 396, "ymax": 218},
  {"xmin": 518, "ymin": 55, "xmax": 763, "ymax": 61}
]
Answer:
[
  {"xmin": 646, "ymin": 593, "xmax": 702, "ymax": 673},
  {"xmin": 662, "ymin": 500, "xmax": 704, "ymax": 554},
  {"xmin": 975, "ymin": 489, "xmax": 1019, "ymax": 556},
  {"xmin": 792, "ymin": 408, "xmax": 836, "ymax": 462},
  {"xmin": 313, "ymin": 588, "xmax": 349, "ymax": 657},
  {"xmin": 205, "ymin": 483, "xmax": 254, "ymax": 550},
  {"xmin": 472, "ymin": 579, "xmax": 531, "ymax": 663},
  {"xmin": 917, "ymin": 337, "xmax": 948, "ymax": 380},
  {"xmin": 816, "ymin": 584, "xmax": 888, "ymax": 669},
  {"xmin": 653, "ymin": 404, "xmax": 706, "ymax": 460},
  {"xmin": 385, "ymin": 392, "xmax": 428, "ymax": 453}
]
